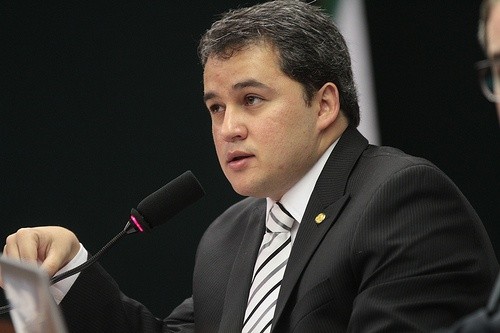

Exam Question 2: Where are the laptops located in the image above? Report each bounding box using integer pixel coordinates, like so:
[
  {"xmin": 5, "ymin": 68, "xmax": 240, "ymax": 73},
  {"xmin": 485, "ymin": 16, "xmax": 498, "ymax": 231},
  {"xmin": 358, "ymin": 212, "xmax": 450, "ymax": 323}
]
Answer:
[{"xmin": 0, "ymin": 258, "xmax": 67, "ymax": 333}]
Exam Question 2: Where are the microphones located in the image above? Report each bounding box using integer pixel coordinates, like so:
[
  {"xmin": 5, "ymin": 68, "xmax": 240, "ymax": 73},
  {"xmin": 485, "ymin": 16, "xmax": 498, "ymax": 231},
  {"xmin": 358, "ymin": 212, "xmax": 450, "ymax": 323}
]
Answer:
[{"xmin": 0, "ymin": 169, "xmax": 205, "ymax": 314}]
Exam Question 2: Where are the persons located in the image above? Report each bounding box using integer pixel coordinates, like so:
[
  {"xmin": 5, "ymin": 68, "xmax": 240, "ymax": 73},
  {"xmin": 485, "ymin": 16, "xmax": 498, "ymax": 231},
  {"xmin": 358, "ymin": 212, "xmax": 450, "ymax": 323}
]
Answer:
[
  {"xmin": 478, "ymin": 0, "xmax": 500, "ymax": 123},
  {"xmin": 0, "ymin": 1, "xmax": 500, "ymax": 333}
]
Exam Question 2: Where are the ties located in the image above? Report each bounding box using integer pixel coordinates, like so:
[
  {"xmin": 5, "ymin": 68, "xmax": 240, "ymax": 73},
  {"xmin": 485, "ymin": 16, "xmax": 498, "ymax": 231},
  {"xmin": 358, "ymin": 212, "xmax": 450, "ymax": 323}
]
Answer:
[{"xmin": 239, "ymin": 201, "xmax": 295, "ymax": 333}]
